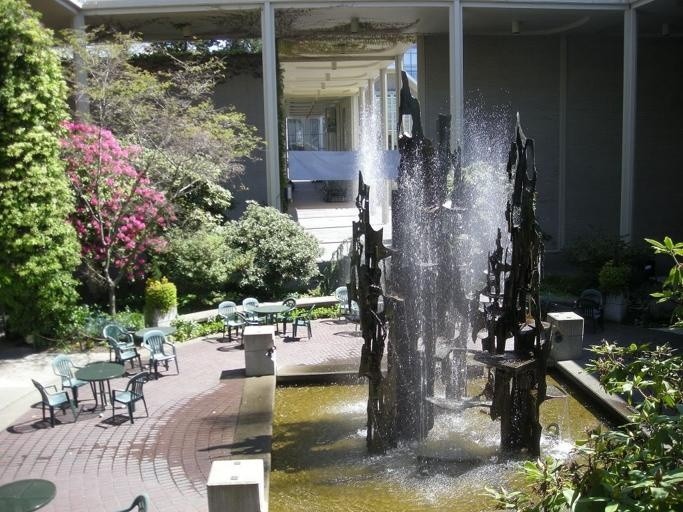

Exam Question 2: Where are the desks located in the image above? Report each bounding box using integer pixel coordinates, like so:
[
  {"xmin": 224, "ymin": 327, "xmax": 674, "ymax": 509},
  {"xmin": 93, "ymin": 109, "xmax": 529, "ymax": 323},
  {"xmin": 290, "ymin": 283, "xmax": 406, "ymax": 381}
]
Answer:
[
  {"xmin": 74, "ymin": 362, "xmax": 126, "ymax": 410},
  {"xmin": 134, "ymin": 326, "xmax": 175, "ymax": 367},
  {"xmin": 0, "ymin": 479, "xmax": 57, "ymax": 512},
  {"xmin": 253, "ymin": 305, "xmax": 290, "ymax": 335}
]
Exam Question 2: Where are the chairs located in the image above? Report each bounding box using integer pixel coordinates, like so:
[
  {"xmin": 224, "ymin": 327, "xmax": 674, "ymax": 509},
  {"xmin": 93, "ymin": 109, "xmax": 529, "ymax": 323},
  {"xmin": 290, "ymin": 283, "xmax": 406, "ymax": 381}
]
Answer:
[
  {"xmin": 242, "ymin": 298, "xmax": 266, "ymax": 326},
  {"xmin": 112, "ymin": 371, "xmax": 149, "ymax": 424},
  {"xmin": 52, "ymin": 354, "xmax": 96, "ymax": 408},
  {"xmin": 31, "ymin": 378, "xmax": 80, "ymax": 428},
  {"xmin": 118, "ymin": 495, "xmax": 149, "ymax": 512},
  {"xmin": 102, "ymin": 323, "xmax": 143, "ymax": 377},
  {"xmin": 292, "ymin": 304, "xmax": 316, "ymax": 340},
  {"xmin": 143, "ymin": 330, "xmax": 179, "ymax": 380},
  {"xmin": 271, "ymin": 298, "xmax": 297, "ymax": 335},
  {"xmin": 335, "ymin": 286, "xmax": 354, "ymax": 321},
  {"xmin": 218, "ymin": 301, "xmax": 247, "ymax": 342}
]
